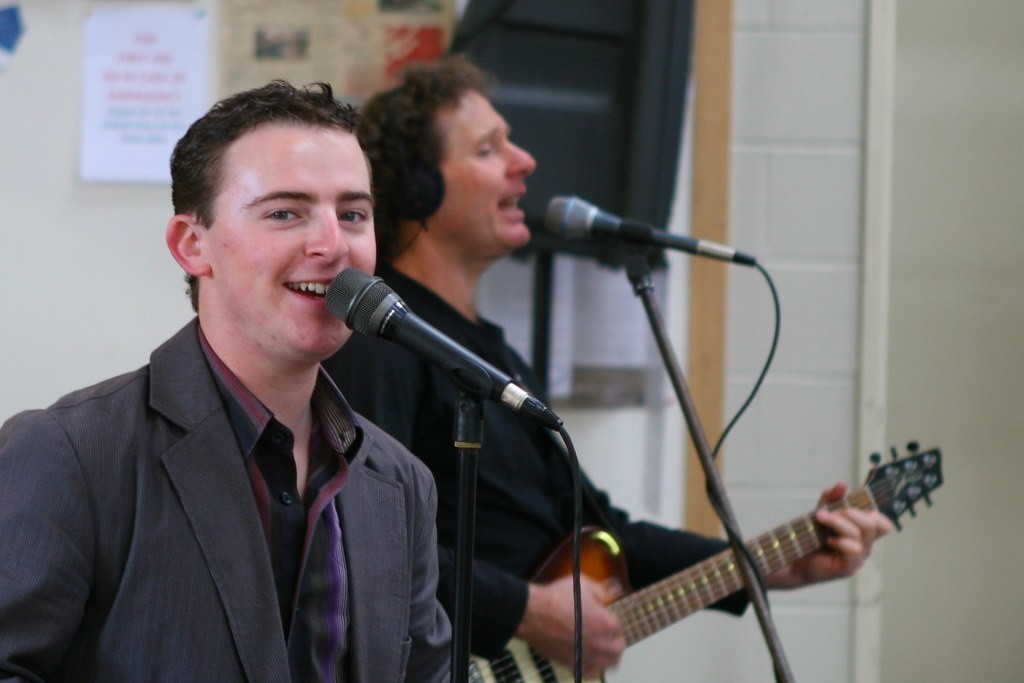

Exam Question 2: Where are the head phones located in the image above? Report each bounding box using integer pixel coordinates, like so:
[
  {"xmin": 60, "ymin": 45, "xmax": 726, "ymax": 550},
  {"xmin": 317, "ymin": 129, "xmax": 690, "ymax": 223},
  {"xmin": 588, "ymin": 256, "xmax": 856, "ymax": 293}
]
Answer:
[{"xmin": 381, "ymin": 87, "xmax": 447, "ymax": 231}]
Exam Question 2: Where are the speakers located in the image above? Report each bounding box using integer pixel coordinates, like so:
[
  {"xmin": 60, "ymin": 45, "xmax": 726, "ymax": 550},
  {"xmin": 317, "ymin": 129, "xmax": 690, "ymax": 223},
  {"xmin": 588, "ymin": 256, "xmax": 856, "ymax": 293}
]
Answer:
[{"xmin": 449, "ymin": 0, "xmax": 698, "ymax": 262}]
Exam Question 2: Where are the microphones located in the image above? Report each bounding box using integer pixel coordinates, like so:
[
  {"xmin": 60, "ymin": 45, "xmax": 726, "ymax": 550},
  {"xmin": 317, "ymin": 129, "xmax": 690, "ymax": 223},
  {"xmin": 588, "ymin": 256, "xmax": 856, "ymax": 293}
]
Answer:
[
  {"xmin": 544, "ymin": 194, "xmax": 757, "ymax": 267},
  {"xmin": 325, "ymin": 267, "xmax": 562, "ymax": 432}
]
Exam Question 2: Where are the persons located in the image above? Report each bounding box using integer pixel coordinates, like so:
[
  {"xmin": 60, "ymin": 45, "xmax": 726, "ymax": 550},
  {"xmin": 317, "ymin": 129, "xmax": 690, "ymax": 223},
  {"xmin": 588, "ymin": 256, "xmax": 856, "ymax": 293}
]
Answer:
[
  {"xmin": 0, "ymin": 79, "xmax": 451, "ymax": 683},
  {"xmin": 326, "ymin": 61, "xmax": 893, "ymax": 676}
]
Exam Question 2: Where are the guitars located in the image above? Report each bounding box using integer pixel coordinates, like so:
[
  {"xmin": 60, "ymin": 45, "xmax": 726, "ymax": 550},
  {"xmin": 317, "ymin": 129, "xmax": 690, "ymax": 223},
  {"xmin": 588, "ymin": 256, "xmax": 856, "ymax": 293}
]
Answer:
[{"xmin": 462, "ymin": 439, "xmax": 945, "ymax": 683}]
